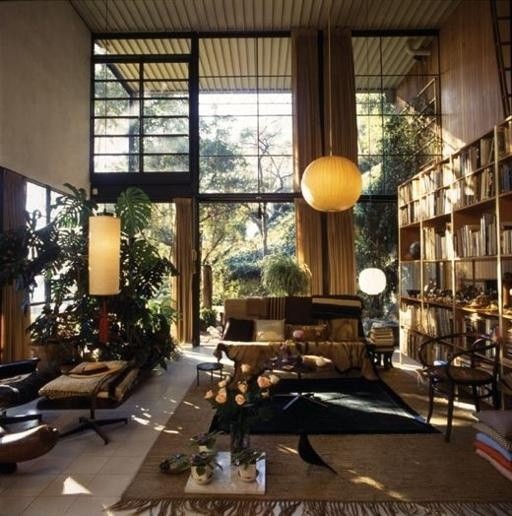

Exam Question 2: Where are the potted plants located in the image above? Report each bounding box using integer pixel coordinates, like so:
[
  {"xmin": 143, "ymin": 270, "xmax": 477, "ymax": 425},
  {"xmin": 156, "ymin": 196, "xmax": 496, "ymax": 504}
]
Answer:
[
  {"xmin": 0, "ymin": 182, "xmax": 99, "ymax": 377},
  {"xmin": 232, "ymin": 446, "xmax": 266, "ymax": 482}
]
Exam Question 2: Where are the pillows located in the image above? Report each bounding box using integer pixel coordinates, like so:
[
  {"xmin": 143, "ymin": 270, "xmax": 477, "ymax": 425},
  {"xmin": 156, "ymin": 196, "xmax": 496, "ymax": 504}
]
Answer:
[
  {"xmin": 222, "ymin": 317, "xmax": 359, "ymax": 343},
  {"xmin": 68, "ymin": 361, "xmax": 110, "ymax": 375},
  {"xmin": 300, "ymin": 354, "xmax": 333, "ymax": 367}
]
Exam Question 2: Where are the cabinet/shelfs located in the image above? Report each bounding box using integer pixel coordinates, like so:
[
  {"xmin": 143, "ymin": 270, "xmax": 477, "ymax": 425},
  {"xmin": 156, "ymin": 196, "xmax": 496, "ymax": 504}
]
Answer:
[{"xmin": 397, "ymin": 116, "xmax": 512, "ymax": 412}]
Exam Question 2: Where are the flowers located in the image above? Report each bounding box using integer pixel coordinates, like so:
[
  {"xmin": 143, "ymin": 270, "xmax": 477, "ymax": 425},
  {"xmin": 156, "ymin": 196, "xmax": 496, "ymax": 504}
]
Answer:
[
  {"xmin": 183, "ymin": 429, "xmax": 223, "ymax": 472},
  {"xmin": 267, "ymin": 329, "xmax": 304, "ymax": 367},
  {"xmin": 202, "ymin": 363, "xmax": 281, "ymax": 447}
]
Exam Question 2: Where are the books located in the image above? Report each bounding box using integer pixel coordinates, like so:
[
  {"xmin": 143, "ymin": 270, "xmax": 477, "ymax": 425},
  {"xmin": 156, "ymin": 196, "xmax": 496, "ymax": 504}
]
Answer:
[{"xmin": 397, "ymin": 122, "xmax": 511, "ymax": 414}]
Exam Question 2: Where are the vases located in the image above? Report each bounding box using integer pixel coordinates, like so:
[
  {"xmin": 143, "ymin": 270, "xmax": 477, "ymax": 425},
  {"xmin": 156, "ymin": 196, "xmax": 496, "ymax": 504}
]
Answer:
[
  {"xmin": 228, "ymin": 424, "xmax": 250, "ymax": 470},
  {"xmin": 190, "ymin": 465, "xmax": 213, "ymax": 485}
]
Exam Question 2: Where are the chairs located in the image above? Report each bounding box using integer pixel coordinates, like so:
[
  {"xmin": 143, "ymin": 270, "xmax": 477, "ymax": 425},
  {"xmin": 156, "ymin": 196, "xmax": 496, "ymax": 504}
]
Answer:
[{"xmin": 416, "ymin": 333, "xmax": 501, "ymax": 443}]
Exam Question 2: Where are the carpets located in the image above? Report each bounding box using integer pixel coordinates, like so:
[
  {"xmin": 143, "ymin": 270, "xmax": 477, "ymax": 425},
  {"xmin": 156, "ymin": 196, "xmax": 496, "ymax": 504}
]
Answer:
[{"xmin": 103, "ymin": 366, "xmax": 512, "ymax": 516}]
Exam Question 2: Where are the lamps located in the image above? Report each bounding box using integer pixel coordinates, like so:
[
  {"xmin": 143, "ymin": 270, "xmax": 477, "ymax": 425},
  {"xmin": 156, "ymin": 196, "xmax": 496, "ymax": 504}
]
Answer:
[
  {"xmin": 85, "ymin": 0, "xmax": 123, "ymax": 297},
  {"xmin": 298, "ymin": 0, "xmax": 364, "ymax": 213}
]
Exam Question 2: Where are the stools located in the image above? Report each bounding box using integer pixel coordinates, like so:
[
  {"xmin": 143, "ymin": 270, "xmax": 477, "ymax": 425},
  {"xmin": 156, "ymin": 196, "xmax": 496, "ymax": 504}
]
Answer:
[
  {"xmin": 0, "ymin": 360, "xmax": 52, "ymax": 424},
  {"xmin": 36, "ymin": 360, "xmax": 145, "ymax": 445},
  {"xmin": 197, "ymin": 363, "xmax": 223, "ymax": 386}
]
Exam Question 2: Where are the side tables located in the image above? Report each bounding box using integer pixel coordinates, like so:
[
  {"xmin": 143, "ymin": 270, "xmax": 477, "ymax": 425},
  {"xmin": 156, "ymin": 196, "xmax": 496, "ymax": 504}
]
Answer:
[{"xmin": 366, "ymin": 337, "xmax": 395, "ymax": 370}]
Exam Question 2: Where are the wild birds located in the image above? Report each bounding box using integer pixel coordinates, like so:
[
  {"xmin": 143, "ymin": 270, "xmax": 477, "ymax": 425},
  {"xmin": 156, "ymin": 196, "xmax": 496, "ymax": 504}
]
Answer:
[{"xmin": 296, "ymin": 428, "xmax": 339, "ymax": 476}]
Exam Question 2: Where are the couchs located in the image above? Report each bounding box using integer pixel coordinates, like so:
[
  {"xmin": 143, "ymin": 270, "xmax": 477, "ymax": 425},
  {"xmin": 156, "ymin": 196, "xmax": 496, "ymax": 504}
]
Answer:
[{"xmin": 218, "ymin": 294, "xmax": 367, "ymax": 378}]
